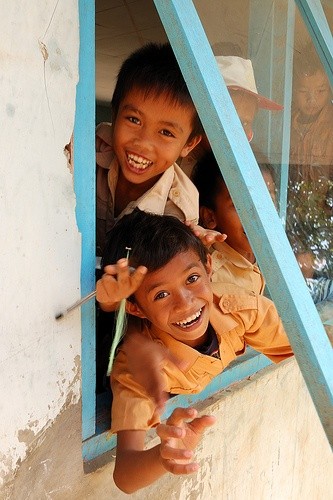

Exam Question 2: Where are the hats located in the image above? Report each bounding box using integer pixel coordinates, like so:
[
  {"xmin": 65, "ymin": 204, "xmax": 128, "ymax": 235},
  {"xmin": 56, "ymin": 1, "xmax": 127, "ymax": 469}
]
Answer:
[{"xmin": 214, "ymin": 55, "xmax": 285, "ymax": 111}]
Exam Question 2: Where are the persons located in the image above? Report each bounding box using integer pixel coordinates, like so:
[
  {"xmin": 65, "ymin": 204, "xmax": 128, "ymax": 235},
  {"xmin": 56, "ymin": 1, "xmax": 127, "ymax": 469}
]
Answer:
[
  {"xmin": 108, "ymin": 216, "xmax": 333, "ymax": 496},
  {"xmin": 177, "ymin": 35, "xmax": 332, "ymax": 303},
  {"xmin": 94, "ymin": 40, "xmax": 204, "ymax": 313}
]
